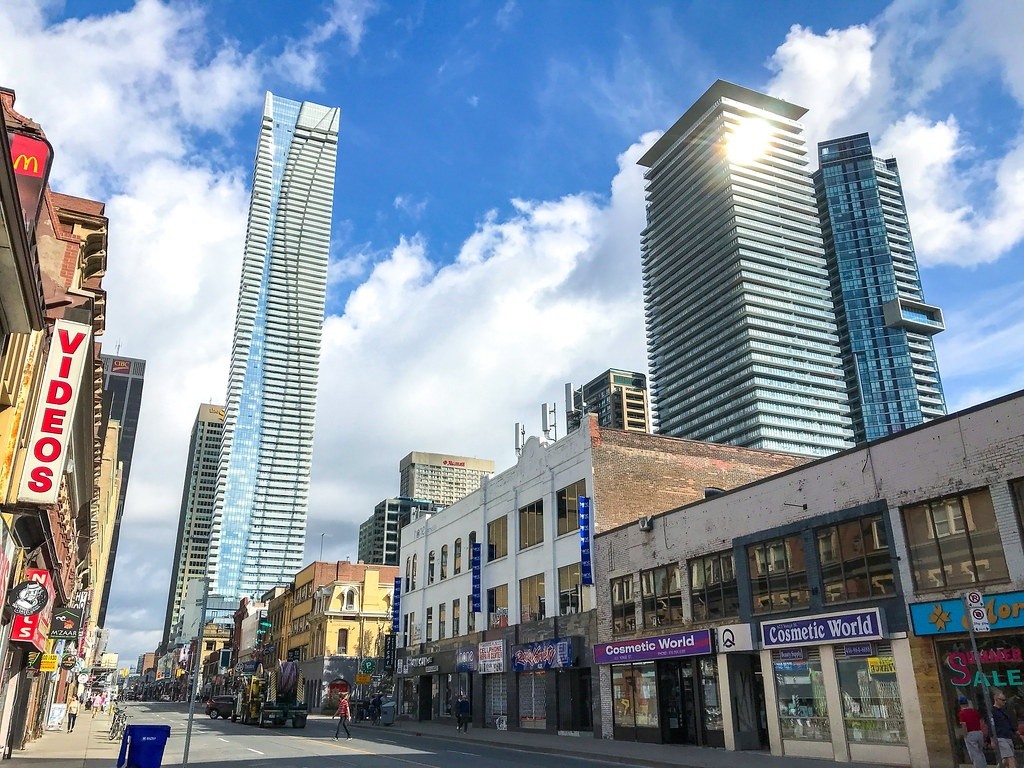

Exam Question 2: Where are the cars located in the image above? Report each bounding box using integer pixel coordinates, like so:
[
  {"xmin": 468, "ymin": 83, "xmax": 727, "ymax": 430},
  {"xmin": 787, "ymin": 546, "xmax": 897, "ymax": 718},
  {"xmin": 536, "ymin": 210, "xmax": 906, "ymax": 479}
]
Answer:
[
  {"xmin": 126, "ymin": 691, "xmax": 144, "ymax": 700},
  {"xmin": 158, "ymin": 694, "xmax": 171, "ymax": 702},
  {"xmin": 204, "ymin": 695, "xmax": 237, "ymax": 719}
]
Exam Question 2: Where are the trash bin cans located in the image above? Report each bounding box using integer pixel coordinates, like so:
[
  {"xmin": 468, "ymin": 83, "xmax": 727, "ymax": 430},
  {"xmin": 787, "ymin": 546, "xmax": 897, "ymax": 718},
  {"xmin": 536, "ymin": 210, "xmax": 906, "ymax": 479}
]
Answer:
[
  {"xmin": 116, "ymin": 725, "xmax": 171, "ymax": 768},
  {"xmin": 380, "ymin": 701, "xmax": 397, "ymax": 727}
]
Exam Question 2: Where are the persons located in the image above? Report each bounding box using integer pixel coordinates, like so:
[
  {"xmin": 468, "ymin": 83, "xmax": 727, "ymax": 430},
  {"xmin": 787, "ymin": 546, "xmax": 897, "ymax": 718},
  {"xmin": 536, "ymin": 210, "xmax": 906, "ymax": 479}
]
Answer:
[
  {"xmin": 332, "ymin": 694, "xmax": 352, "ymax": 741},
  {"xmin": 64, "ymin": 692, "xmax": 150, "ymax": 733},
  {"xmin": 958, "ymin": 695, "xmax": 990, "ymax": 768},
  {"xmin": 362, "ymin": 695, "xmax": 389, "ymax": 726},
  {"xmin": 453, "ymin": 695, "xmax": 470, "ymax": 734},
  {"xmin": 991, "ymin": 689, "xmax": 1024, "ymax": 768}
]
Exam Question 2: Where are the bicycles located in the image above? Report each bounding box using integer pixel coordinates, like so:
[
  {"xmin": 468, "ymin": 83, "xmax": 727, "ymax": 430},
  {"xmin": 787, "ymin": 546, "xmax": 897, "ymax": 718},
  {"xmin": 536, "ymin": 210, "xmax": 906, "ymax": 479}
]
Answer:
[{"xmin": 108, "ymin": 701, "xmax": 134, "ymax": 741}]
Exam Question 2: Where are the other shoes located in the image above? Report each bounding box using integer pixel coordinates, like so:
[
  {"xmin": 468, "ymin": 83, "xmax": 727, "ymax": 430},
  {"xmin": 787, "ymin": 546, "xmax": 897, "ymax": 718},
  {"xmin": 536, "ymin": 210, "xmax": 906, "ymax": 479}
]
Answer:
[
  {"xmin": 346, "ymin": 737, "xmax": 353, "ymax": 742},
  {"xmin": 464, "ymin": 731, "xmax": 469, "ymax": 734},
  {"xmin": 67, "ymin": 730, "xmax": 69, "ymax": 733},
  {"xmin": 332, "ymin": 736, "xmax": 338, "ymax": 741},
  {"xmin": 70, "ymin": 730, "xmax": 73, "ymax": 732}
]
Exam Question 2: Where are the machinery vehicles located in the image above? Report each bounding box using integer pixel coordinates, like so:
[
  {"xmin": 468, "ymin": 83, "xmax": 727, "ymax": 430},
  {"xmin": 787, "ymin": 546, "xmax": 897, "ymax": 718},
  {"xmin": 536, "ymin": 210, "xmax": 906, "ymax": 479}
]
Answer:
[{"xmin": 230, "ymin": 658, "xmax": 309, "ymax": 729}]
